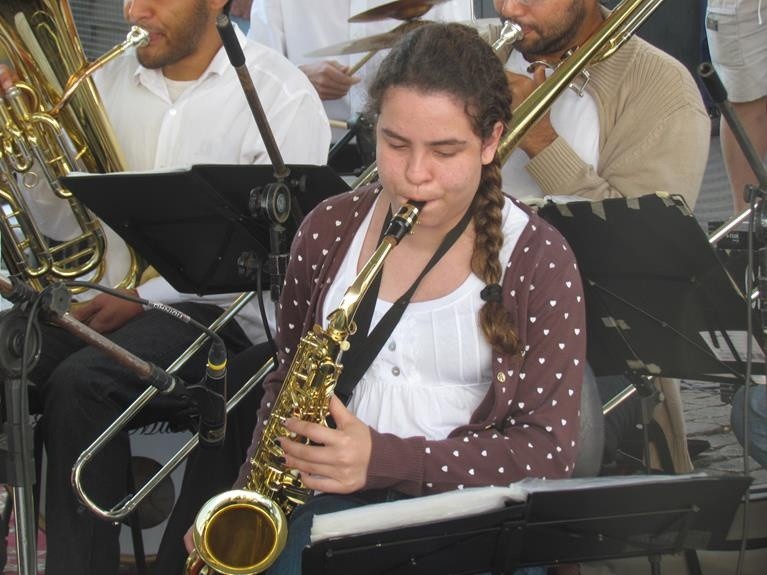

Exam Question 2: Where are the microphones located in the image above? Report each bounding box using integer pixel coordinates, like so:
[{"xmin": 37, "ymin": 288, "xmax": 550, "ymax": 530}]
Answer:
[{"xmin": 200, "ymin": 343, "xmax": 227, "ymax": 449}]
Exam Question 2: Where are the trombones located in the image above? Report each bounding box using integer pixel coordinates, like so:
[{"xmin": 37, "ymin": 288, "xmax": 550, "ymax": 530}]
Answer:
[{"xmin": 70, "ymin": 0, "xmax": 665, "ymax": 522}]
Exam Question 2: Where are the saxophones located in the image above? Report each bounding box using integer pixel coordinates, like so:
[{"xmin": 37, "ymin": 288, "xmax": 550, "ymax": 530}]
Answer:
[{"xmin": 182, "ymin": 200, "xmax": 427, "ymax": 575}]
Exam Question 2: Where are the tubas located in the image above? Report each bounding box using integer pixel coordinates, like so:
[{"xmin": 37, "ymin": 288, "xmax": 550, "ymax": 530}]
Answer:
[{"xmin": 0, "ymin": 0, "xmax": 151, "ymax": 328}]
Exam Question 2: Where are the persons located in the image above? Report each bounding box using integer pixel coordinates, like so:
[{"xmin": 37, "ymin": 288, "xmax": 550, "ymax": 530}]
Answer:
[
  {"xmin": 241, "ymin": 1, "xmax": 476, "ymax": 187},
  {"xmin": 179, "ymin": 20, "xmax": 587, "ymax": 575},
  {"xmin": 692, "ymin": 0, "xmax": 767, "ymax": 476},
  {"xmin": 480, "ymin": 0, "xmax": 713, "ymax": 479},
  {"xmin": 0, "ymin": 1, "xmax": 333, "ymax": 573}
]
